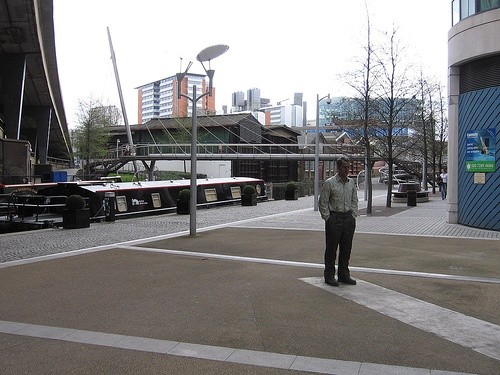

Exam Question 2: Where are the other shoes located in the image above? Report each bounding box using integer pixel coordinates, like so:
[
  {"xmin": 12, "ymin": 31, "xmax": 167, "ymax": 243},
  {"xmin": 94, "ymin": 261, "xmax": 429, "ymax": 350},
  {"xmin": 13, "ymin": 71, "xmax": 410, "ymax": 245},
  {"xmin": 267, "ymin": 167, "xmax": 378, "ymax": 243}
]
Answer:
[
  {"xmin": 325, "ymin": 276, "xmax": 339, "ymax": 286},
  {"xmin": 338, "ymin": 276, "xmax": 356, "ymax": 285}
]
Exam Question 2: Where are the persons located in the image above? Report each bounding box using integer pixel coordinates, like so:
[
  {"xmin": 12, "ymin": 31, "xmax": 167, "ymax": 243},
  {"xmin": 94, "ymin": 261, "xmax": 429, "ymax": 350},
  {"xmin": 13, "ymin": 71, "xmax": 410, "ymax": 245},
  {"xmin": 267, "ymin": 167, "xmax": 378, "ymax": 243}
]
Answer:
[
  {"xmin": 318, "ymin": 156, "xmax": 359, "ymax": 286},
  {"xmin": 437, "ymin": 168, "xmax": 448, "ymax": 200}
]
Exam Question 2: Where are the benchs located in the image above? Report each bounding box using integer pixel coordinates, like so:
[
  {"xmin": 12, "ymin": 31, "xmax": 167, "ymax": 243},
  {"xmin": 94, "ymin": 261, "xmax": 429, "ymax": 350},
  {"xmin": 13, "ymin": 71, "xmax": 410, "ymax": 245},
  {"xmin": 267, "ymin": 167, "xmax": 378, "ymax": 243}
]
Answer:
[{"xmin": 391, "ymin": 190, "xmax": 430, "ymax": 202}]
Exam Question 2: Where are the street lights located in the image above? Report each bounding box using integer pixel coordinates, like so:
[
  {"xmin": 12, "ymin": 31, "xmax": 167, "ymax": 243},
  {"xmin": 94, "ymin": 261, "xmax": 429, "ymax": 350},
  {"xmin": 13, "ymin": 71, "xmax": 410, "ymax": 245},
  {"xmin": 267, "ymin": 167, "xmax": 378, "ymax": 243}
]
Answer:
[
  {"xmin": 176, "ymin": 43, "xmax": 229, "ymax": 235},
  {"xmin": 314, "ymin": 93, "xmax": 332, "ymax": 210}
]
